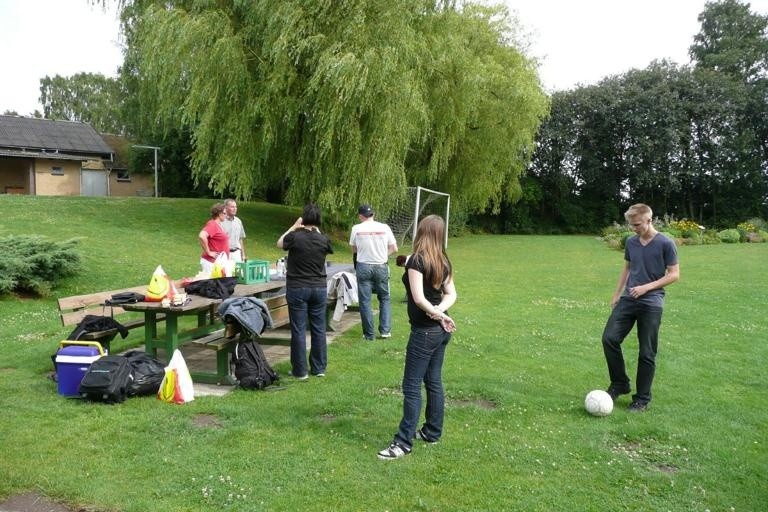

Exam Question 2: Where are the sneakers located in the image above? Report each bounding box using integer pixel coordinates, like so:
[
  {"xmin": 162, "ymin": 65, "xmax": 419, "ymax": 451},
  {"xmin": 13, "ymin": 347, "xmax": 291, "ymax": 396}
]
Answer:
[
  {"xmin": 380, "ymin": 332, "xmax": 391, "ymax": 337},
  {"xmin": 412, "ymin": 431, "xmax": 438, "ymax": 444},
  {"xmin": 606, "ymin": 382, "xmax": 631, "ymax": 399},
  {"xmin": 377, "ymin": 440, "xmax": 412, "ymax": 460},
  {"xmin": 362, "ymin": 334, "xmax": 375, "ymax": 342},
  {"xmin": 316, "ymin": 371, "xmax": 325, "ymax": 377},
  {"xmin": 627, "ymin": 403, "xmax": 648, "ymax": 412},
  {"xmin": 288, "ymin": 370, "xmax": 309, "ymax": 382}
]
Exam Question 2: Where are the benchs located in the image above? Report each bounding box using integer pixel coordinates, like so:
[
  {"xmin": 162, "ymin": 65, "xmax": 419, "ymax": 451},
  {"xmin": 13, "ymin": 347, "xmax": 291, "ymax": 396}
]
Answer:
[
  {"xmin": 190, "ymin": 294, "xmax": 291, "ymax": 385},
  {"xmin": 56, "ymin": 278, "xmax": 197, "ymax": 355}
]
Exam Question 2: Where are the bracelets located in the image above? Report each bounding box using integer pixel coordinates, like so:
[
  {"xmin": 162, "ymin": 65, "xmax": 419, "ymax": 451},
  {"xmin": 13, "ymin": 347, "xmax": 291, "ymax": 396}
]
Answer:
[{"xmin": 440, "ymin": 312, "xmax": 445, "ymax": 321}]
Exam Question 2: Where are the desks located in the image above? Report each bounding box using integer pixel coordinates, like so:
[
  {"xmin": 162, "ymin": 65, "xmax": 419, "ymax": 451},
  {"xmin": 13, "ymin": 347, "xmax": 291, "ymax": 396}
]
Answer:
[{"xmin": 118, "ymin": 279, "xmax": 289, "ymax": 380}]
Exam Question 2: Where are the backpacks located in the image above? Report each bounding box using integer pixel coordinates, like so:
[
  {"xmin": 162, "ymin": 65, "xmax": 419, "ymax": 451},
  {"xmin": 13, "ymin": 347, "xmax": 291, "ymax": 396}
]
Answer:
[
  {"xmin": 125, "ymin": 350, "xmax": 166, "ymax": 399},
  {"xmin": 50, "ymin": 314, "xmax": 129, "ymax": 372},
  {"xmin": 78, "ymin": 355, "xmax": 135, "ymax": 405},
  {"xmin": 184, "ymin": 277, "xmax": 237, "ymax": 300},
  {"xmin": 229, "ymin": 339, "xmax": 279, "ymax": 391}
]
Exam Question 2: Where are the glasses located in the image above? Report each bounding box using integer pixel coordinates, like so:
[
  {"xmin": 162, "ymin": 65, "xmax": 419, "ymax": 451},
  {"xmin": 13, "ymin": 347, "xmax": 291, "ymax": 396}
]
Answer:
[{"xmin": 222, "ymin": 213, "xmax": 226, "ymax": 215}]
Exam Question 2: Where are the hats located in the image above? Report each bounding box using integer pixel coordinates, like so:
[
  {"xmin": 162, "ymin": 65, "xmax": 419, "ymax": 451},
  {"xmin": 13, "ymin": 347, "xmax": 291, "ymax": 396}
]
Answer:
[{"xmin": 358, "ymin": 205, "xmax": 374, "ymax": 214}]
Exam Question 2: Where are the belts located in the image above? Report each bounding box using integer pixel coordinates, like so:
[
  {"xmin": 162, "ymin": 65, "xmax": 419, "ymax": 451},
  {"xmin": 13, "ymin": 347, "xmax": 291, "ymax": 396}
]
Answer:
[{"xmin": 230, "ymin": 248, "xmax": 239, "ymax": 252}]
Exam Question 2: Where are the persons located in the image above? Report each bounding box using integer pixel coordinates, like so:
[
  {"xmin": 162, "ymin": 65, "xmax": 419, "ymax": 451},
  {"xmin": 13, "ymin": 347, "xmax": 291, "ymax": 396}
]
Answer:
[
  {"xmin": 220, "ymin": 197, "xmax": 248, "ymax": 267},
  {"xmin": 348, "ymin": 203, "xmax": 399, "ymax": 340},
  {"xmin": 602, "ymin": 201, "xmax": 681, "ymax": 414},
  {"xmin": 198, "ymin": 202, "xmax": 231, "ymax": 275},
  {"xmin": 276, "ymin": 205, "xmax": 334, "ymax": 380},
  {"xmin": 375, "ymin": 214, "xmax": 457, "ymax": 462},
  {"xmin": 395, "ymin": 254, "xmax": 412, "ymax": 268}
]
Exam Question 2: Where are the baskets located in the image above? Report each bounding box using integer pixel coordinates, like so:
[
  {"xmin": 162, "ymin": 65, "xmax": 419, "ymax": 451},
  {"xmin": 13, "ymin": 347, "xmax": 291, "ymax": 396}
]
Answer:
[{"xmin": 235, "ymin": 260, "xmax": 270, "ymax": 285}]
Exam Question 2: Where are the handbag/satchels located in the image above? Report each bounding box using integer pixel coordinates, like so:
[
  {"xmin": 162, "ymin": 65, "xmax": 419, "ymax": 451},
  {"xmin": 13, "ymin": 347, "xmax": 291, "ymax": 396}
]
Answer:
[{"xmin": 106, "ymin": 291, "xmax": 144, "ymax": 304}]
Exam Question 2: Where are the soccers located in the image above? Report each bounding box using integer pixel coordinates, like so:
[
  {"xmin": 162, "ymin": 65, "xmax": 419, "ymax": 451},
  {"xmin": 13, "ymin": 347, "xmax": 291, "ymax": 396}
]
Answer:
[{"xmin": 585, "ymin": 390, "xmax": 613, "ymax": 417}]
